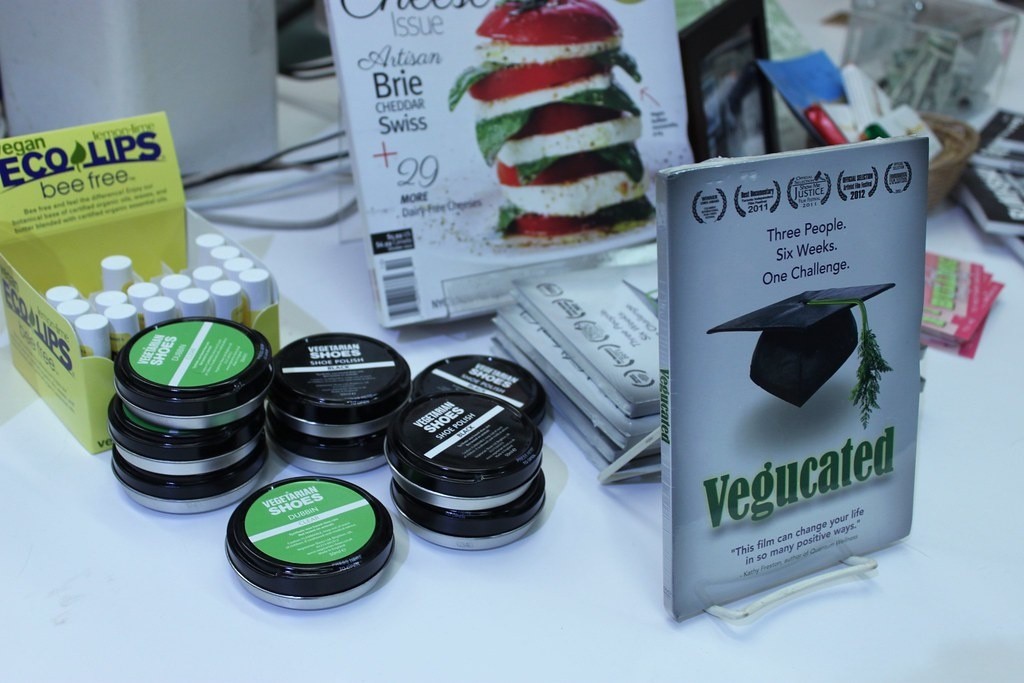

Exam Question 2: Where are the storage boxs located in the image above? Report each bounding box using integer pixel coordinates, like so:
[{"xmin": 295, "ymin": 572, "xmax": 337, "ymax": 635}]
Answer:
[{"xmin": 0, "ymin": 111, "xmax": 281, "ymax": 453}]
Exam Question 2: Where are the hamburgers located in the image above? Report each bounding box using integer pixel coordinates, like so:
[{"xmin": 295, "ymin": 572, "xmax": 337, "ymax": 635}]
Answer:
[{"xmin": 447, "ymin": 0, "xmax": 659, "ymax": 248}]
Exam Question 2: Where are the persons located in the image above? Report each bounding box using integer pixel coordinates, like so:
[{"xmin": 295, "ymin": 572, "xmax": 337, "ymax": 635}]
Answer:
[{"xmin": 723, "ymin": 75, "xmax": 768, "ymax": 161}]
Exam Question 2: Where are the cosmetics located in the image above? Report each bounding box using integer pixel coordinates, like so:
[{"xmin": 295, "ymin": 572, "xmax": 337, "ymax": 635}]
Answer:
[{"xmin": 110, "ymin": 313, "xmax": 551, "ymax": 609}]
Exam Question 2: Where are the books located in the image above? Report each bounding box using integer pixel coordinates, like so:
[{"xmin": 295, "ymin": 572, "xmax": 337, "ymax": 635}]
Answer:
[
  {"xmin": 325, "ymin": 0, "xmax": 701, "ymax": 328},
  {"xmin": 654, "ymin": 133, "xmax": 933, "ymax": 621},
  {"xmin": 488, "ymin": 259, "xmax": 663, "ymax": 484},
  {"xmin": 953, "ymin": 103, "xmax": 1023, "ymax": 262}
]
